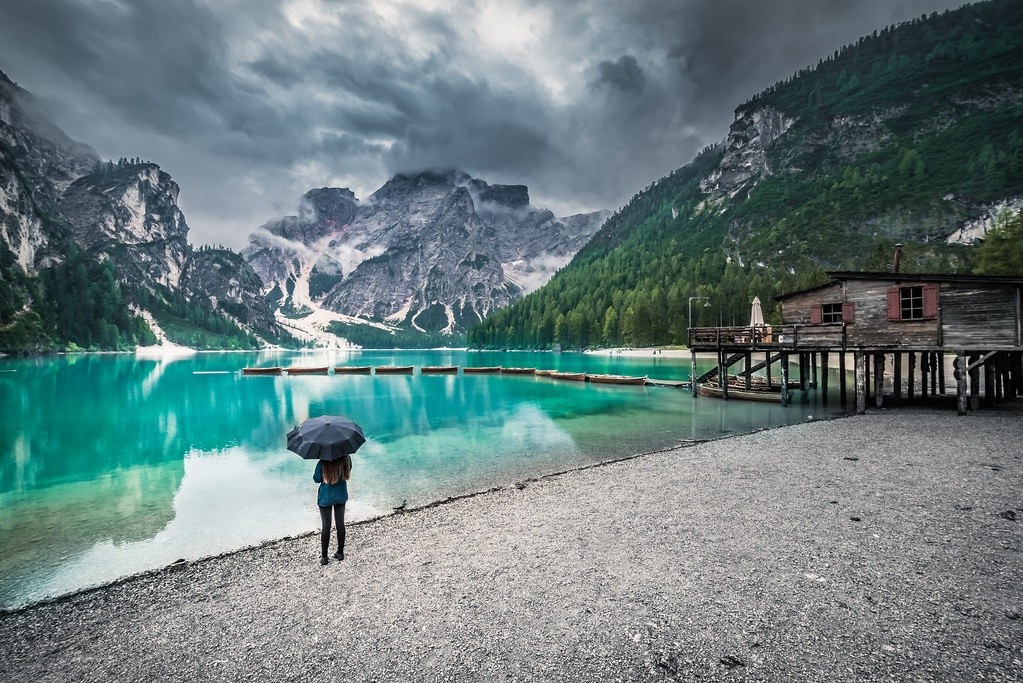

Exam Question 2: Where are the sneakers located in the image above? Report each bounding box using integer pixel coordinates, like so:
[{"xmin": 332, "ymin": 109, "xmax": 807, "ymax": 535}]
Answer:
[
  {"xmin": 332, "ymin": 551, "xmax": 344, "ymax": 561},
  {"xmin": 319, "ymin": 555, "xmax": 328, "ymax": 564}
]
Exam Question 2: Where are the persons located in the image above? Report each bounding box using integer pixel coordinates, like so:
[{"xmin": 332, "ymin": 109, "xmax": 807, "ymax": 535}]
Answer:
[{"xmin": 313, "ymin": 454, "xmax": 353, "ymax": 565}]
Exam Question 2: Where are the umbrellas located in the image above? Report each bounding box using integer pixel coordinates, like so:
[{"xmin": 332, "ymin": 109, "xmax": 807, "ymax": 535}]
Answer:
[
  {"xmin": 286, "ymin": 414, "xmax": 366, "ymax": 460},
  {"xmin": 750, "ymin": 296, "xmax": 764, "ymax": 326}
]
